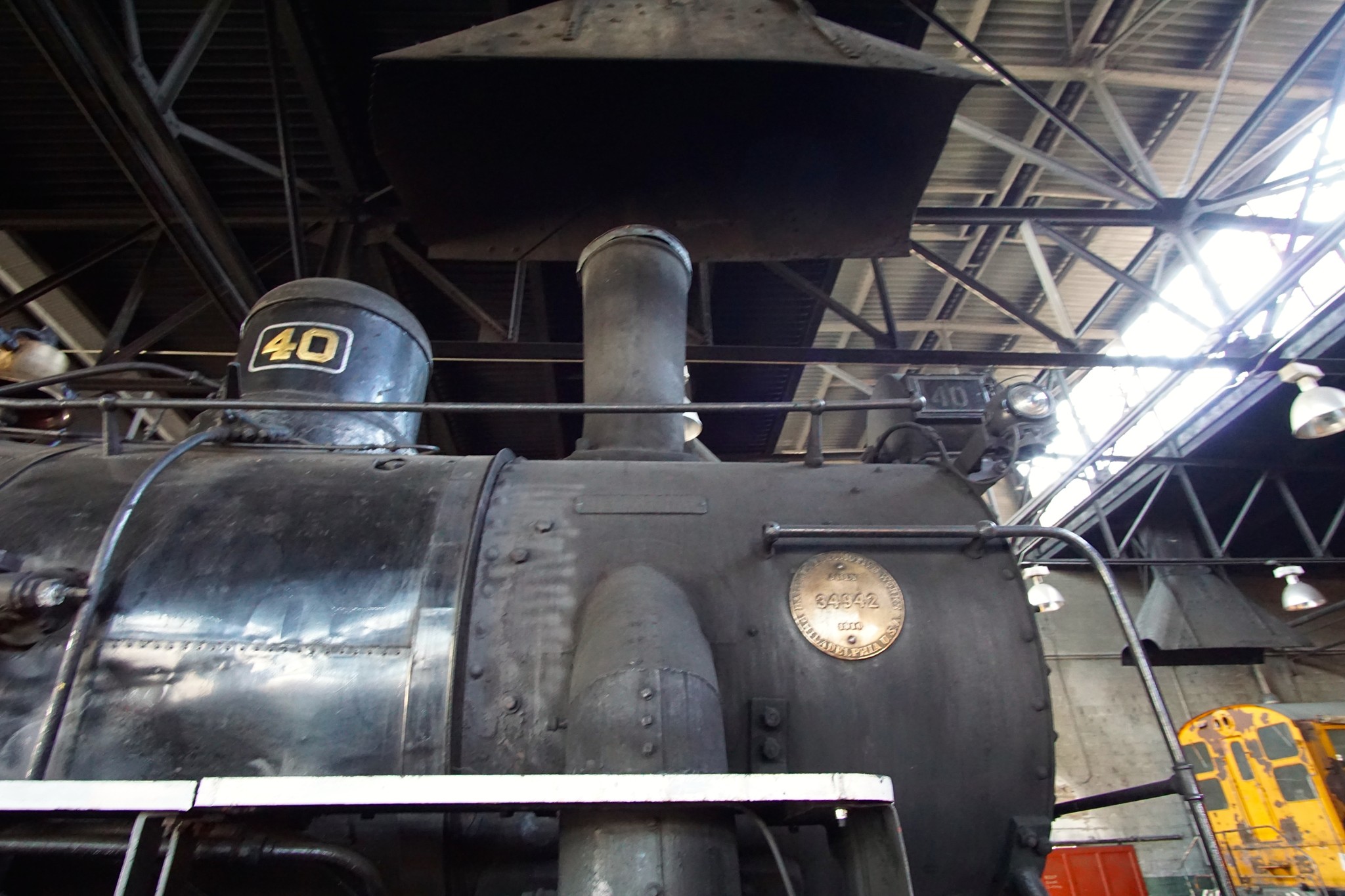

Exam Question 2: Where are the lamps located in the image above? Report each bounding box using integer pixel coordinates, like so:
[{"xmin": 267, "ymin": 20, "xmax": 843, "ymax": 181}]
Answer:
[
  {"xmin": 1277, "ymin": 362, "xmax": 1345, "ymax": 439},
  {"xmin": 1272, "ymin": 565, "xmax": 1327, "ymax": 611},
  {"xmin": 1021, "ymin": 566, "xmax": 1065, "ymax": 613}
]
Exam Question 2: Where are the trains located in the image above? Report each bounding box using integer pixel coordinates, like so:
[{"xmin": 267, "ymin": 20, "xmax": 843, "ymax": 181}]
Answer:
[{"xmin": 0, "ymin": 222, "xmax": 1241, "ymax": 896}]
